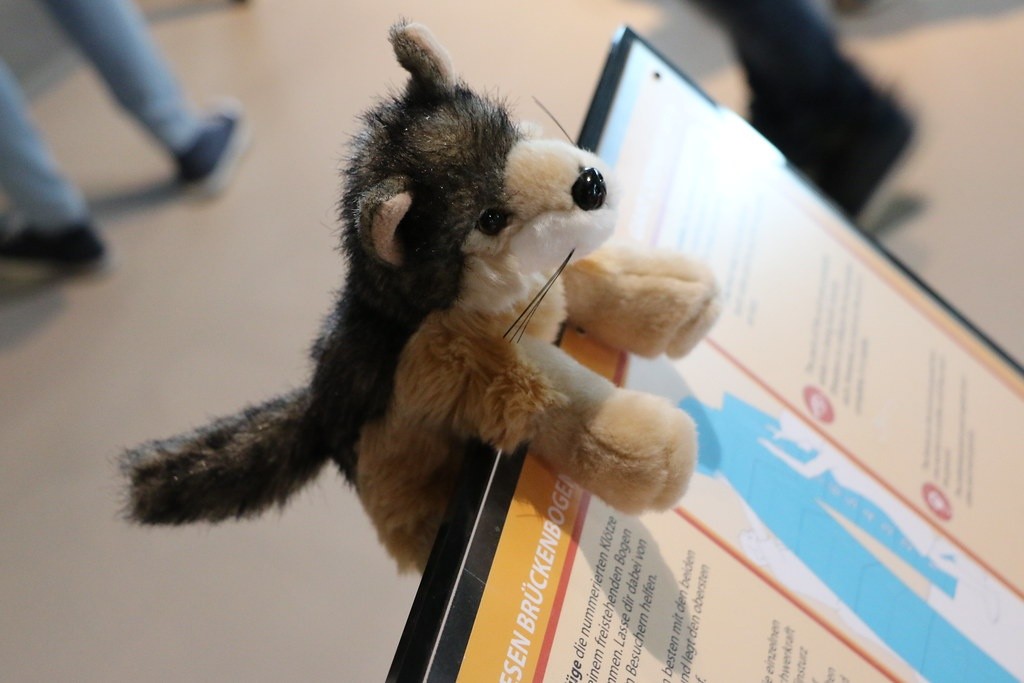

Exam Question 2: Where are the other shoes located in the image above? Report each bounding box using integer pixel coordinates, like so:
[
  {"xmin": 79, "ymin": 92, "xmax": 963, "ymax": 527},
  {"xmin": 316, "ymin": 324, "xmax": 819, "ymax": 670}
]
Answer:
[
  {"xmin": 178, "ymin": 107, "xmax": 247, "ymax": 184},
  {"xmin": 12, "ymin": 223, "xmax": 101, "ymax": 269},
  {"xmin": 809, "ymin": 103, "xmax": 918, "ymax": 214}
]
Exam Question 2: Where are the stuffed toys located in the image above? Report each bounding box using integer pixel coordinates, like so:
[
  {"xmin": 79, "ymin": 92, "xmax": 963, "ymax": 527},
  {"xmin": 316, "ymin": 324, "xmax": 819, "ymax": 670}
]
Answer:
[{"xmin": 120, "ymin": 22, "xmax": 723, "ymax": 572}]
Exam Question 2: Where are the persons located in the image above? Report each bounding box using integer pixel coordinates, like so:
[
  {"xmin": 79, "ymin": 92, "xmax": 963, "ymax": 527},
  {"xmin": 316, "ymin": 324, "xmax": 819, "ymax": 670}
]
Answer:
[
  {"xmin": 692, "ymin": 0, "xmax": 913, "ymax": 217},
  {"xmin": 0, "ymin": 0, "xmax": 250, "ymax": 278}
]
